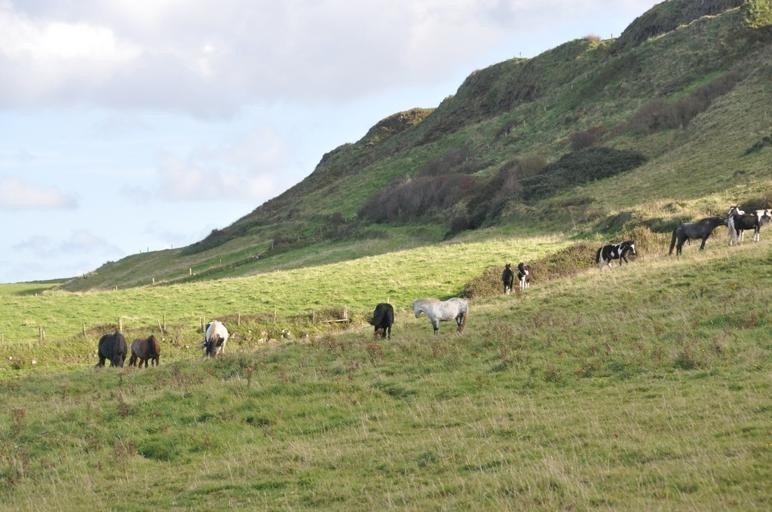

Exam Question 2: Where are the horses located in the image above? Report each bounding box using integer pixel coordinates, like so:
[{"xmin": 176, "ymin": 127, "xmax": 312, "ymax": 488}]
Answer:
[
  {"xmin": 412, "ymin": 296, "xmax": 468, "ymax": 337},
  {"xmin": 502, "ymin": 263, "xmax": 532, "ymax": 295},
  {"xmin": 203, "ymin": 320, "xmax": 230, "ymax": 361},
  {"xmin": 367, "ymin": 303, "xmax": 395, "ymax": 340},
  {"xmin": 596, "ymin": 240, "xmax": 636, "ymax": 276},
  {"xmin": 669, "ymin": 204, "xmax": 772, "ymax": 256},
  {"xmin": 96, "ymin": 332, "xmax": 160, "ymax": 368}
]
